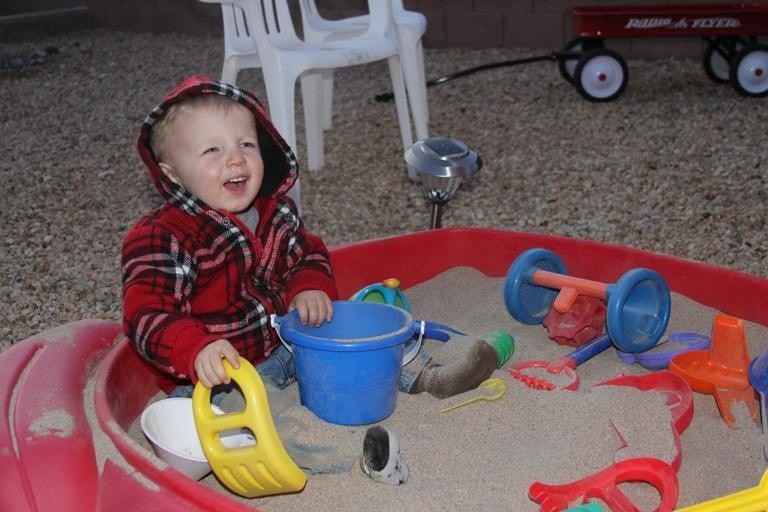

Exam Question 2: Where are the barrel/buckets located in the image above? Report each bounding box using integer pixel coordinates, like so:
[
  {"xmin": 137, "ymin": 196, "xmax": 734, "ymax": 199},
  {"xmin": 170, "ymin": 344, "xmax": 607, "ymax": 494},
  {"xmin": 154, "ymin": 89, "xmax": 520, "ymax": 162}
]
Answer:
[{"xmin": 269, "ymin": 298, "xmax": 425, "ymax": 428}]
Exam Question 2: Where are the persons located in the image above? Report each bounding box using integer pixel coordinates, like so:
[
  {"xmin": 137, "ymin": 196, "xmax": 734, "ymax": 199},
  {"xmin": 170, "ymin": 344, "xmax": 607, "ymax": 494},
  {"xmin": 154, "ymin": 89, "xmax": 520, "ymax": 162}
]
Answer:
[{"xmin": 120, "ymin": 75, "xmax": 496, "ymax": 484}]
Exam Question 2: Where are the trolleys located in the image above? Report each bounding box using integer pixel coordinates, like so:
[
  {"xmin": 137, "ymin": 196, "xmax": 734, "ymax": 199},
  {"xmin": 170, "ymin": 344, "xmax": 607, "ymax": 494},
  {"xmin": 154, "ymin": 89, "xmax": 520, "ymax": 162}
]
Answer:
[{"xmin": 376, "ymin": 6, "xmax": 768, "ymax": 102}]
